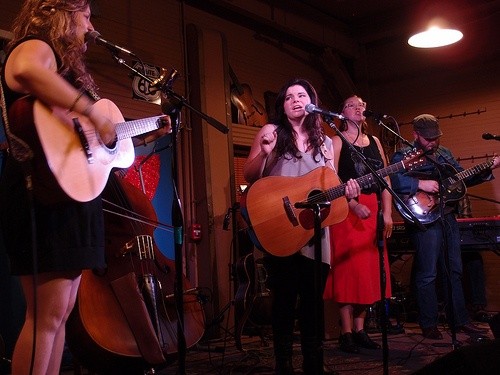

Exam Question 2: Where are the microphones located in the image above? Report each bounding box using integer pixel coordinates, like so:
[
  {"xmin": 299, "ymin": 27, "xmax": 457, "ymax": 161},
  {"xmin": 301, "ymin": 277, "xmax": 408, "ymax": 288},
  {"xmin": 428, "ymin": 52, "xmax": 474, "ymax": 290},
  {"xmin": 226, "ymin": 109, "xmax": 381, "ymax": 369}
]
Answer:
[
  {"xmin": 165, "ymin": 287, "xmax": 201, "ymax": 298},
  {"xmin": 363, "ymin": 111, "xmax": 390, "ymax": 119},
  {"xmin": 482, "ymin": 133, "xmax": 500, "ymax": 141},
  {"xmin": 86, "ymin": 31, "xmax": 137, "ymax": 57},
  {"xmin": 305, "ymin": 104, "xmax": 348, "ymax": 120}
]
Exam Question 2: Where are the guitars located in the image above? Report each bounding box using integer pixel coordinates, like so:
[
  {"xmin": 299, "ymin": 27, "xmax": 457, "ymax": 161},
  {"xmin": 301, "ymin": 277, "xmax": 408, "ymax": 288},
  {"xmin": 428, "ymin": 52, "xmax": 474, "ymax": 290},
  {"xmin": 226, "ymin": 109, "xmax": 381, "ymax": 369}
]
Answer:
[
  {"xmin": 239, "ymin": 147, "xmax": 429, "ymax": 259},
  {"xmin": 2, "ymin": 94, "xmax": 184, "ymax": 204},
  {"xmin": 392, "ymin": 153, "xmax": 500, "ymax": 225}
]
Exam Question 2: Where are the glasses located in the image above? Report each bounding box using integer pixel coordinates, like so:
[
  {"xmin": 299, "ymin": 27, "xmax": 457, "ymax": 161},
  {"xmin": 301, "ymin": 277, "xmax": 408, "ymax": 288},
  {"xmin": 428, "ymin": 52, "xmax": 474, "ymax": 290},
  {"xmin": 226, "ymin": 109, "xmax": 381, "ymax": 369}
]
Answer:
[{"xmin": 343, "ymin": 102, "xmax": 366, "ymax": 113}]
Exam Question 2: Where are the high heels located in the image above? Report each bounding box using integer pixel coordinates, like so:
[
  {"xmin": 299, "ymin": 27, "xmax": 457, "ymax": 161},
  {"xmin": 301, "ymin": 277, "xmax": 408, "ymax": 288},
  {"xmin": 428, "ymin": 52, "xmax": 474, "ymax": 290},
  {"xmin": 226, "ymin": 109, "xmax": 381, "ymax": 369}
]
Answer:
[
  {"xmin": 352, "ymin": 328, "xmax": 381, "ymax": 350},
  {"xmin": 340, "ymin": 332, "xmax": 360, "ymax": 354}
]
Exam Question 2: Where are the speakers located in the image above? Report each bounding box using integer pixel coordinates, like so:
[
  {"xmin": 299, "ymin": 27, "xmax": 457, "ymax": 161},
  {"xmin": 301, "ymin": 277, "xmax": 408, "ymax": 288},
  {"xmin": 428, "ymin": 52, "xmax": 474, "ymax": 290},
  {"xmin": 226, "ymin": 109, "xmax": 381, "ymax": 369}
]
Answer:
[{"xmin": 412, "ymin": 312, "xmax": 500, "ymax": 375}]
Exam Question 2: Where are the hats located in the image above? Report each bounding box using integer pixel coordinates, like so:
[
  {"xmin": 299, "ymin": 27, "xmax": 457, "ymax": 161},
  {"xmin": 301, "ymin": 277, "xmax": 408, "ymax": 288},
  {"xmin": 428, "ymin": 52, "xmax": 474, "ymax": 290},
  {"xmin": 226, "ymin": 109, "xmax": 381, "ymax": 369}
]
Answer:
[{"xmin": 413, "ymin": 113, "xmax": 443, "ymax": 139}]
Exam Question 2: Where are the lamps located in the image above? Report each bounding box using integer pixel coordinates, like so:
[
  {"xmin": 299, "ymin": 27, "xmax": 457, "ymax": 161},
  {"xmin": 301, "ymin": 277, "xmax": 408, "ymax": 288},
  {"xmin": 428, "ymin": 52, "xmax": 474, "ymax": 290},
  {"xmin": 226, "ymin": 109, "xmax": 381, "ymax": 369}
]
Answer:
[{"xmin": 408, "ymin": 15, "xmax": 464, "ymax": 48}]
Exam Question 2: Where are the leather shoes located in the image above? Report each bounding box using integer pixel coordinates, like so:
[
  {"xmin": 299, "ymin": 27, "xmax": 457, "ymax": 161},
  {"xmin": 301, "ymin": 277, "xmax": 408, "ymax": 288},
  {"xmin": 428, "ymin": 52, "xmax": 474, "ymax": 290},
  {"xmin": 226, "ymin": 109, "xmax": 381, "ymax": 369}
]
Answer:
[
  {"xmin": 452, "ymin": 322, "xmax": 487, "ymax": 334},
  {"xmin": 423, "ymin": 326, "xmax": 443, "ymax": 339}
]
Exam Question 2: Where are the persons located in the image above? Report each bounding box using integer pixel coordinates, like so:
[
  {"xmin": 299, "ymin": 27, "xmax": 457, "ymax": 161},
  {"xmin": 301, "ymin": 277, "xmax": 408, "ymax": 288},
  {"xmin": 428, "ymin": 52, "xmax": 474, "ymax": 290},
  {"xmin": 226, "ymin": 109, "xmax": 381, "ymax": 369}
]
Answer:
[
  {"xmin": 244, "ymin": 79, "xmax": 363, "ymax": 375},
  {"xmin": 0, "ymin": 0, "xmax": 172, "ymax": 375},
  {"xmin": 325, "ymin": 91, "xmax": 394, "ymax": 354},
  {"xmin": 392, "ymin": 113, "xmax": 500, "ymax": 339}
]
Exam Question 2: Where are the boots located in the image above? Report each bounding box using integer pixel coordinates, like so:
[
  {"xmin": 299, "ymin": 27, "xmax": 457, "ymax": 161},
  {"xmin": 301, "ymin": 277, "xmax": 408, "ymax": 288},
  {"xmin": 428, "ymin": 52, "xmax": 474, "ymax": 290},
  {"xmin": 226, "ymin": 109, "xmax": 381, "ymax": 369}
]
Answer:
[
  {"xmin": 303, "ymin": 341, "xmax": 325, "ymax": 374},
  {"xmin": 273, "ymin": 341, "xmax": 297, "ymax": 375}
]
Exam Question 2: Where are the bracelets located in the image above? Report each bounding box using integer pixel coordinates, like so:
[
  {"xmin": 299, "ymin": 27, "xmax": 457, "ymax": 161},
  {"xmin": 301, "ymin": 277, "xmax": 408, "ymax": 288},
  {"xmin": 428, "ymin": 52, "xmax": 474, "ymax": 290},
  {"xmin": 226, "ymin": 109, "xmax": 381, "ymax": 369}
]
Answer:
[{"xmin": 354, "ymin": 202, "xmax": 359, "ymax": 211}]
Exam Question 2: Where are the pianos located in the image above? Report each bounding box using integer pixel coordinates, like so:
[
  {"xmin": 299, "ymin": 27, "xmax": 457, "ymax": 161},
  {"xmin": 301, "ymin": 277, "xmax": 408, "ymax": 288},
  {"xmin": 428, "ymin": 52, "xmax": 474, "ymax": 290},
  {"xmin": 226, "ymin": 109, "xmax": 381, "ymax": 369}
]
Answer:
[{"xmin": 363, "ymin": 215, "xmax": 500, "ymax": 334}]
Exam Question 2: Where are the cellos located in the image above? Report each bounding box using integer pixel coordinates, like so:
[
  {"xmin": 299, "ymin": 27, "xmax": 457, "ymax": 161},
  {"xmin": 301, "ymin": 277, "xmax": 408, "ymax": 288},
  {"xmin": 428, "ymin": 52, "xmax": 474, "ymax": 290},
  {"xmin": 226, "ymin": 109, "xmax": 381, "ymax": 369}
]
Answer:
[
  {"xmin": 64, "ymin": 168, "xmax": 208, "ymax": 375},
  {"xmin": 233, "ymin": 244, "xmax": 274, "ymax": 338}
]
